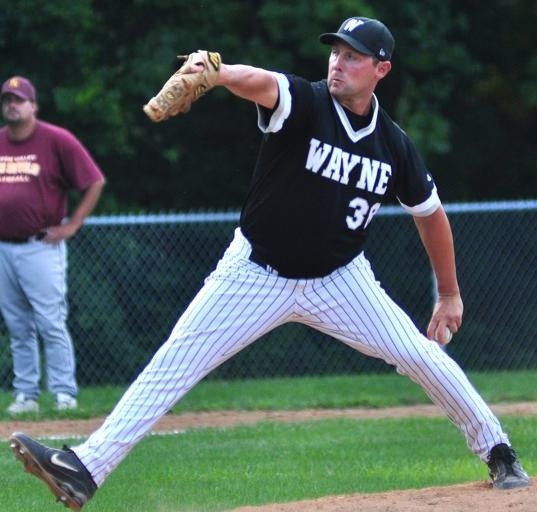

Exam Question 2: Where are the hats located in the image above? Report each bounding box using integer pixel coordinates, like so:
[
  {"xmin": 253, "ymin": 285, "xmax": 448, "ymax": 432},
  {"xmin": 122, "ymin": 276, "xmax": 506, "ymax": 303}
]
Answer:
[
  {"xmin": 1, "ymin": 76, "xmax": 35, "ymax": 101},
  {"xmin": 320, "ymin": 17, "xmax": 395, "ymax": 61}
]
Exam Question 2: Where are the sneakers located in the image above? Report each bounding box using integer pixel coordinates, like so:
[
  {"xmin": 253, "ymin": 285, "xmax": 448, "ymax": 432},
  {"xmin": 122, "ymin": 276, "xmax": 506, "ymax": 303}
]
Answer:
[
  {"xmin": 487, "ymin": 443, "xmax": 529, "ymax": 489},
  {"xmin": 9, "ymin": 431, "xmax": 98, "ymax": 512},
  {"xmin": 7, "ymin": 393, "xmax": 38, "ymax": 413},
  {"xmin": 56, "ymin": 393, "xmax": 77, "ymax": 409}
]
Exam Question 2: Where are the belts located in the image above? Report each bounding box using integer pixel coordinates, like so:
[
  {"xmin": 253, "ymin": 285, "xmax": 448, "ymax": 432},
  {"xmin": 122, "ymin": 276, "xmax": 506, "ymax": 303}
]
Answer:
[{"xmin": 0, "ymin": 232, "xmax": 47, "ymax": 244}]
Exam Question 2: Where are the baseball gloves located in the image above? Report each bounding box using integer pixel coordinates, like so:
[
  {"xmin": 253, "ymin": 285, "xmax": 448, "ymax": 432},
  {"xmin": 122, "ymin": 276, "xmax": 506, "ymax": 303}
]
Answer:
[{"xmin": 143, "ymin": 49, "xmax": 221, "ymax": 122}]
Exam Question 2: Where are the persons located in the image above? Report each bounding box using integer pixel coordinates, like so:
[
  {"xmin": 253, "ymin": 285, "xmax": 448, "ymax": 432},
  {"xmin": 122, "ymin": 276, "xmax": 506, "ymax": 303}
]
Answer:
[
  {"xmin": 1, "ymin": 76, "xmax": 106, "ymax": 414},
  {"xmin": 8, "ymin": 17, "xmax": 531, "ymax": 512}
]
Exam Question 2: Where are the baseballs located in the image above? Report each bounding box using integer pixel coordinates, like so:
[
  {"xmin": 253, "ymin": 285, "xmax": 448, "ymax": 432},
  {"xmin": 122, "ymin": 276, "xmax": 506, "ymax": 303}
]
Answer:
[{"xmin": 434, "ymin": 325, "xmax": 454, "ymax": 344}]
